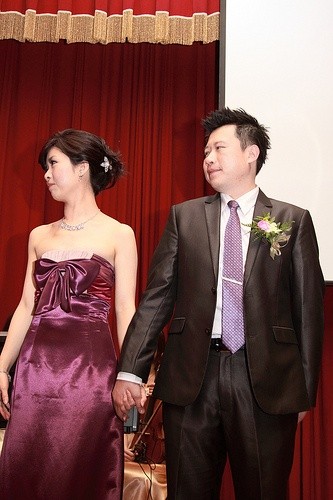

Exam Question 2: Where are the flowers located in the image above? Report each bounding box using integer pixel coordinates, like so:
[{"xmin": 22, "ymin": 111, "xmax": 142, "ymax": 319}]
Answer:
[{"xmin": 241, "ymin": 209, "xmax": 296, "ymax": 260}]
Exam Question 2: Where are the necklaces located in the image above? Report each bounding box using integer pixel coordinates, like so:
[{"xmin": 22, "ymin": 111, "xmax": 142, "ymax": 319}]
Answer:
[{"xmin": 60, "ymin": 207, "xmax": 101, "ymax": 231}]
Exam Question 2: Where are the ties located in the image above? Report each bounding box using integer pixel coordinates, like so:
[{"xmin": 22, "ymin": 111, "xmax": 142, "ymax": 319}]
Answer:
[{"xmin": 222, "ymin": 200, "xmax": 247, "ymax": 354}]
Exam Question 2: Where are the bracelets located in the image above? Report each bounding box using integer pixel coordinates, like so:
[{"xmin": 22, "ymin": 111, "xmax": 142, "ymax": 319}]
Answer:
[{"xmin": 0, "ymin": 370, "xmax": 12, "ymax": 382}]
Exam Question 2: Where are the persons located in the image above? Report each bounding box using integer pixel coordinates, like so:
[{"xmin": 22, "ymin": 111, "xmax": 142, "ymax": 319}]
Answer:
[
  {"xmin": 110, "ymin": 109, "xmax": 324, "ymax": 500},
  {"xmin": 0, "ymin": 129, "xmax": 147, "ymax": 500},
  {"xmin": 122, "ymin": 433, "xmax": 168, "ymax": 500}
]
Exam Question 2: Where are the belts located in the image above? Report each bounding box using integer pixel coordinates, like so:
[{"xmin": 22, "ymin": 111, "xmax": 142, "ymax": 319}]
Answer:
[{"xmin": 211, "ymin": 339, "xmax": 246, "ymax": 351}]
